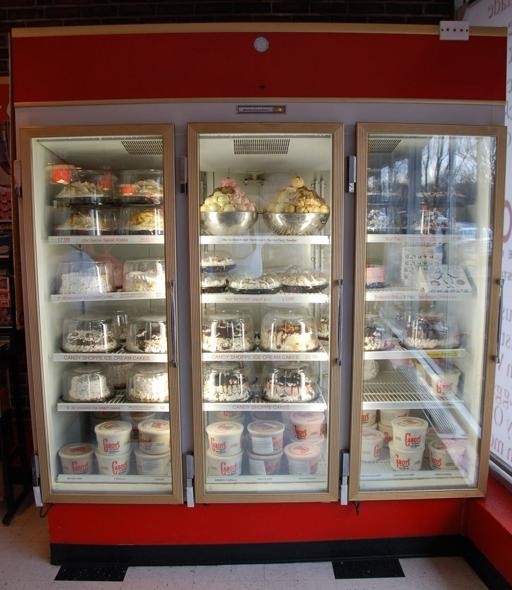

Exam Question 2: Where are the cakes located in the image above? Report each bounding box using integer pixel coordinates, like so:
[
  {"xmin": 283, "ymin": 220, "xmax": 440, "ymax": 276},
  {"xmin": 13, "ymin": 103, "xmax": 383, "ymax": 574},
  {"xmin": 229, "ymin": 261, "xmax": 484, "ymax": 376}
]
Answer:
[
  {"xmin": 202, "ymin": 371, "xmax": 250, "ymax": 404},
  {"xmin": 200, "ymin": 321, "xmax": 255, "ymax": 351},
  {"xmin": 261, "ymin": 321, "xmax": 318, "ymax": 351},
  {"xmin": 281, "ymin": 267, "xmax": 327, "ymax": 288},
  {"xmin": 134, "ymin": 328, "xmax": 166, "ymax": 354},
  {"xmin": 132, "ymin": 370, "xmax": 168, "ymax": 401},
  {"xmin": 65, "ymin": 322, "xmax": 118, "ymax": 353},
  {"xmin": 200, "ymin": 254, "xmax": 236, "ymax": 292},
  {"xmin": 403, "ymin": 319, "xmax": 457, "ymax": 349},
  {"xmin": 57, "ymin": 269, "xmax": 114, "ymax": 296},
  {"xmin": 230, "ymin": 275, "xmax": 280, "ymax": 293},
  {"xmin": 261, "ymin": 375, "xmax": 318, "ymax": 403},
  {"xmin": 364, "ymin": 327, "xmax": 391, "ymax": 348},
  {"xmin": 122, "ymin": 268, "xmax": 168, "ymax": 293},
  {"xmin": 68, "ymin": 373, "xmax": 111, "ymax": 401}
]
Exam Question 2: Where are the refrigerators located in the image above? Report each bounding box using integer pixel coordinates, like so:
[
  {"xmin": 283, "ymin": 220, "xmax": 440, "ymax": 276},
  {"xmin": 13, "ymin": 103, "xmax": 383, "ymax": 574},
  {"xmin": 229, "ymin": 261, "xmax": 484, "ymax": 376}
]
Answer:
[{"xmin": 7, "ymin": 24, "xmax": 509, "ymax": 566}]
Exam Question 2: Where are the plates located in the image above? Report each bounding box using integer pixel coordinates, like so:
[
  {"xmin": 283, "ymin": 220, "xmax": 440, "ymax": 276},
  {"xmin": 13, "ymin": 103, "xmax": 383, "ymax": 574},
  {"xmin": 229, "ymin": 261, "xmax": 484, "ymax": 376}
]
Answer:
[
  {"xmin": 228, "ymin": 284, "xmax": 281, "ymax": 294},
  {"xmin": 282, "ymin": 281, "xmax": 330, "ymax": 293},
  {"xmin": 201, "ymin": 285, "xmax": 227, "ymax": 293},
  {"xmin": 201, "ymin": 263, "xmax": 237, "ymax": 273}
]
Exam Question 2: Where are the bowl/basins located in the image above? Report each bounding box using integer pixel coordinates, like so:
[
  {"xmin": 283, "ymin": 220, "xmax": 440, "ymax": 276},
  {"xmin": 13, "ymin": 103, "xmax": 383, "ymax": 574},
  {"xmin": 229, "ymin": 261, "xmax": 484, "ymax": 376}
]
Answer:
[
  {"xmin": 263, "ymin": 212, "xmax": 330, "ymax": 236},
  {"xmin": 199, "ymin": 210, "xmax": 260, "ymax": 235}
]
